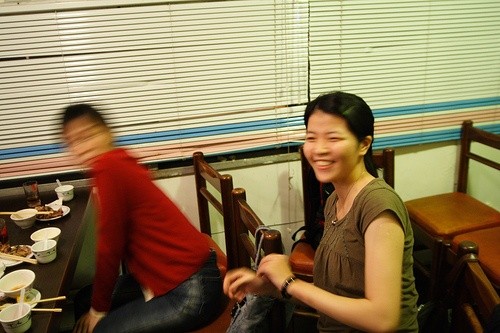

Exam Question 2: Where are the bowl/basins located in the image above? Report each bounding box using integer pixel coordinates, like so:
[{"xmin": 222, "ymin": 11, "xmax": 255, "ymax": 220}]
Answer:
[
  {"xmin": 10, "ymin": 209, "xmax": 38, "ymax": 229},
  {"xmin": 0, "ymin": 269, "xmax": 35, "ymax": 298},
  {"xmin": 30, "ymin": 227, "xmax": 61, "ymax": 243}
]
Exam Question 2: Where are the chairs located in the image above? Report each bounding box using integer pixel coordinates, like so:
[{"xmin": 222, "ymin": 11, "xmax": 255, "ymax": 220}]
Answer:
[{"xmin": 192, "ymin": 119, "xmax": 500, "ymax": 333}]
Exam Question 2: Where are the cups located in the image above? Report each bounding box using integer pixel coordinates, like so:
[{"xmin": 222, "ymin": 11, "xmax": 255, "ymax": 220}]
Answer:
[
  {"xmin": 31, "ymin": 240, "xmax": 57, "ymax": 264},
  {"xmin": 22, "ymin": 181, "xmax": 41, "ymax": 208},
  {"xmin": 55, "ymin": 185, "xmax": 74, "ymax": 201},
  {"xmin": 0, "ymin": 303, "xmax": 32, "ymax": 333}
]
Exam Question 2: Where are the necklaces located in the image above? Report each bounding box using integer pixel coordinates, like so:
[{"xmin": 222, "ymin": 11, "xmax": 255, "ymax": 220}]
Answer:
[{"xmin": 331, "ymin": 168, "xmax": 366, "ymax": 224}]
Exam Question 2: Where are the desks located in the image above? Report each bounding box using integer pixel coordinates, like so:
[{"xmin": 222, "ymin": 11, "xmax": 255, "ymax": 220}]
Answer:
[{"xmin": 0, "ymin": 186, "xmax": 93, "ymax": 333}]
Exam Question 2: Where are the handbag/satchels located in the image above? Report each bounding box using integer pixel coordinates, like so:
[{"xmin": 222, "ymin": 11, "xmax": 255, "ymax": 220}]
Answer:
[
  {"xmin": 227, "ymin": 225, "xmax": 295, "ymax": 333},
  {"xmin": 301, "ymin": 147, "xmax": 333, "ymax": 249}
]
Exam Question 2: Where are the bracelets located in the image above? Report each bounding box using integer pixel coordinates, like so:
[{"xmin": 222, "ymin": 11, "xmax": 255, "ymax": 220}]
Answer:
[{"xmin": 281, "ymin": 276, "xmax": 297, "ymax": 300}]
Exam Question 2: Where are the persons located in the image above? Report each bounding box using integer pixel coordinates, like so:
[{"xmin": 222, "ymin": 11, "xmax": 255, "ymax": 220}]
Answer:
[
  {"xmin": 223, "ymin": 90, "xmax": 419, "ymax": 333},
  {"xmin": 58, "ymin": 102, "xmax": 230, "ymax": 332}
]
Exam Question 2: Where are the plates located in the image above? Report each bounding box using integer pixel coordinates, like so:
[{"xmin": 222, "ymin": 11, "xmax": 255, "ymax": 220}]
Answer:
[
  {"xmin": 0, "ymin": 288, "xmax": 41, "ymax": 308},
  {"xmin": 36, "ymin": 205, "xmax": 70, "ymax": 221},
  {"xmin": 0, "ymin": 245, "xmax": 34, "ymax": 266}
]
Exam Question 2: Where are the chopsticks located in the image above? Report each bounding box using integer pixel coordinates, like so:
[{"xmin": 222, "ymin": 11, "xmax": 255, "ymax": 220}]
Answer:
[
  {"xmin": 0, "ymin": 211, "xmax": 53, "ymax": 214},
  {"xmin": 0, "ymin": 252, "xmax": 37, "ymax": 264},
  {"xmin": 0, "ymin": 296, "xmax": 66, "ymax": 312}
]
